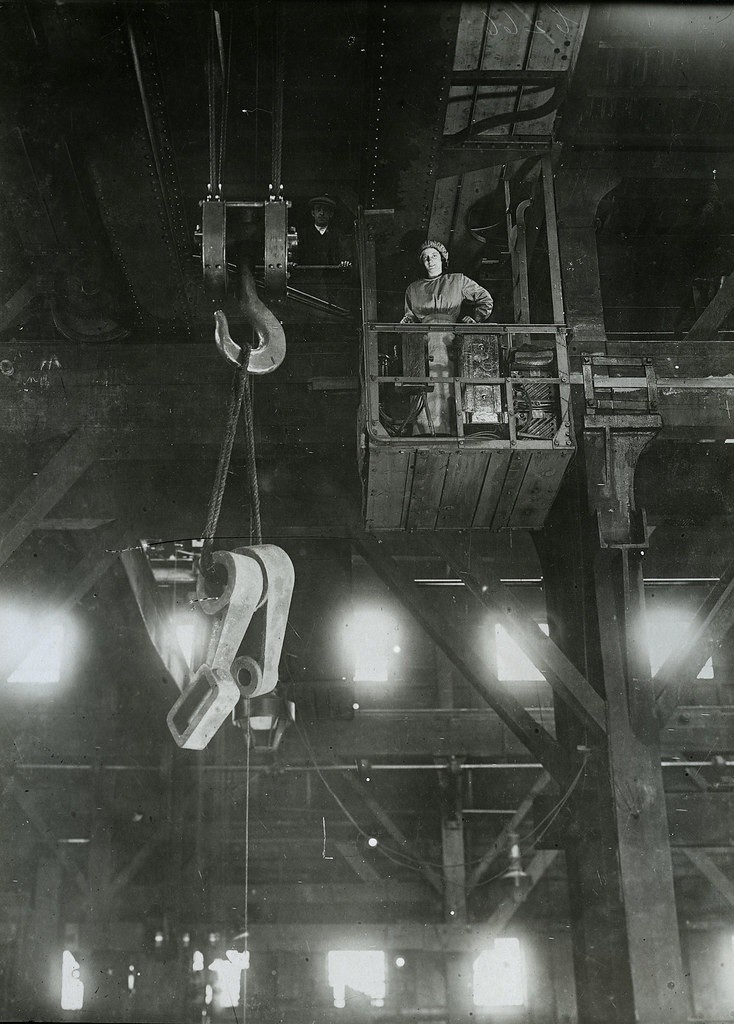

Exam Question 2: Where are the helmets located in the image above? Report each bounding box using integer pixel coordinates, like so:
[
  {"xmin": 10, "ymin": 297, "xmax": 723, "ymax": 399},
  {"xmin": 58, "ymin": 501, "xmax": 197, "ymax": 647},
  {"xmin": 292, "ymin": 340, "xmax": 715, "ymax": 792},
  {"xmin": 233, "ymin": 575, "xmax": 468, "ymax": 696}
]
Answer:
[{"xmin": 417, "ymin": 240, "xmax": 448, "ymax": 264}]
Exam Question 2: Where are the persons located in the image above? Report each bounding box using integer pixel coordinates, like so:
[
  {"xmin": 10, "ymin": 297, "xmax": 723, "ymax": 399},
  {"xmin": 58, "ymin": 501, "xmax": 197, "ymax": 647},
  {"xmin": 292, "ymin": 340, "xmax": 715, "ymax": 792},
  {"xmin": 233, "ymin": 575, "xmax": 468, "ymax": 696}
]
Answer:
[
  {"xmin": 293, "ymin": 196, "xmax": 351, "ymax": 302},
  {"xmin": 403, "ymin": 241, "xmax": 494, "ymax": 436}
]
